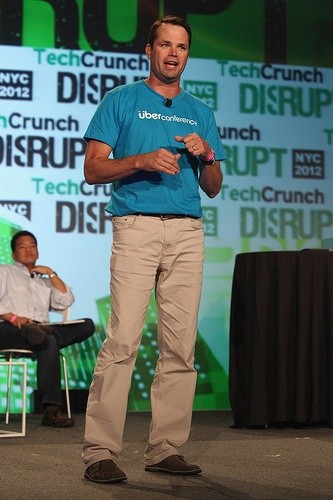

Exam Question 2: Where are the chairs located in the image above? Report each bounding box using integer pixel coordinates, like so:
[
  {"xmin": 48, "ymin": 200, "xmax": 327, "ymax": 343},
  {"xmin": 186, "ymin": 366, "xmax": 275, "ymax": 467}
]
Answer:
[{"xmin": 0, "ymin": 278, "xmax": 70, "ymax": 438}]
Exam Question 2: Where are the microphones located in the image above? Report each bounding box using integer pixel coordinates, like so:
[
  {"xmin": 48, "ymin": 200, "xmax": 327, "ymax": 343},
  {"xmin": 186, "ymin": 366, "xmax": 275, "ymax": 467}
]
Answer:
[
  {"xmin": 165, "ymin": 98, "xmax": 172, "ymax": 107},
  {"xmin": 31, "ymin": 273, "xmax": 34, "ymax": 278}
]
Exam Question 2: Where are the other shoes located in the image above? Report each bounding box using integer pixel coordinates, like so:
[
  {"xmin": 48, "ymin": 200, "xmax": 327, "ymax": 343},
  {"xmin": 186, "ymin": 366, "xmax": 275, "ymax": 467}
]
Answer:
[
  {"xmin": 144, "ymin": 453, "xmax": 203, "ymax": 475},
  {"xmin": 19, "ymin": 323, "xmax": 51, "ymax": 346},
  {"xmin": 83, "ymin": 458, "xmax": 128, "ymax": 484},
  {"xmin": 40, "ymin": 404, "xmax": 75, "ymax": 428}
]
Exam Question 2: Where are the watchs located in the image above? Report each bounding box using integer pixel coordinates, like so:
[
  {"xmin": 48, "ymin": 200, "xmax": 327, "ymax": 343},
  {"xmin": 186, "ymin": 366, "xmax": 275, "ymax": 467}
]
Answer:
[{"xmin": 203, "ymin": 152, "xmax": 215, "ymax": 165}]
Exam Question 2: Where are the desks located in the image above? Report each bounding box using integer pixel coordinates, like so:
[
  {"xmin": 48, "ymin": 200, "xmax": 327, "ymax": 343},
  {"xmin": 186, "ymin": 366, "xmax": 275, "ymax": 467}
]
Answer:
[{"xmin": 229, "ymin": 249, "xmax": 333, "ymax": 429}]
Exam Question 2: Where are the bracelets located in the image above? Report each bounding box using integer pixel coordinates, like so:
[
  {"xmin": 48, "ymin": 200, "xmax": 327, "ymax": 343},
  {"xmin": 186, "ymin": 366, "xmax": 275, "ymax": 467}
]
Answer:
[
  {"xmin": 203, "ymin": 149, "xmax": 213, "ymax": 161},
  {"xmin": 10, "ymin": 314, "xmax": 19, "ymax": 323},
  {"xmin": 50, "ymin": 273, "xmax": 57, "ymax": 278}
]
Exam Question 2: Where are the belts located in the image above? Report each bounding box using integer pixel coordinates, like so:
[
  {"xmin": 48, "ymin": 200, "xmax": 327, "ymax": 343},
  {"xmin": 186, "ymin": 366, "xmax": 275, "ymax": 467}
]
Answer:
[{"xmin": 131, "ymin": 211, "xmax": 188, "ymax": 221}]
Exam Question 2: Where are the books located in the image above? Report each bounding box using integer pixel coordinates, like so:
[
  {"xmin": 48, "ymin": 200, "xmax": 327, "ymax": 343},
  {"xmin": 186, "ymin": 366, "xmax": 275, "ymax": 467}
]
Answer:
[{"xmin": 37, "ymin": 320, "xmax": 85, "ymax": 326}]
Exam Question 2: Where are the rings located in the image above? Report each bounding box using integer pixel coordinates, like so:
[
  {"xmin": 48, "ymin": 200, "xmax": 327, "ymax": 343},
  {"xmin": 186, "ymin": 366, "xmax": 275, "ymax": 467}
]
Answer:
[{"xmin": 192, "ymin": 146, "xmax": 196, "ymax": 151}]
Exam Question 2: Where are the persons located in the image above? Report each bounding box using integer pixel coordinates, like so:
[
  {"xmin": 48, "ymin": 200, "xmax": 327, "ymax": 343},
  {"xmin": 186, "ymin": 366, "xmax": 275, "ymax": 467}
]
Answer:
[
  {"xmin": 0, "ymin": 231, "xmax": 95, "ymax": 428},
  {"xmin": 82, "ymin": 16, "xmax": 227, "ymax": 482}
]
